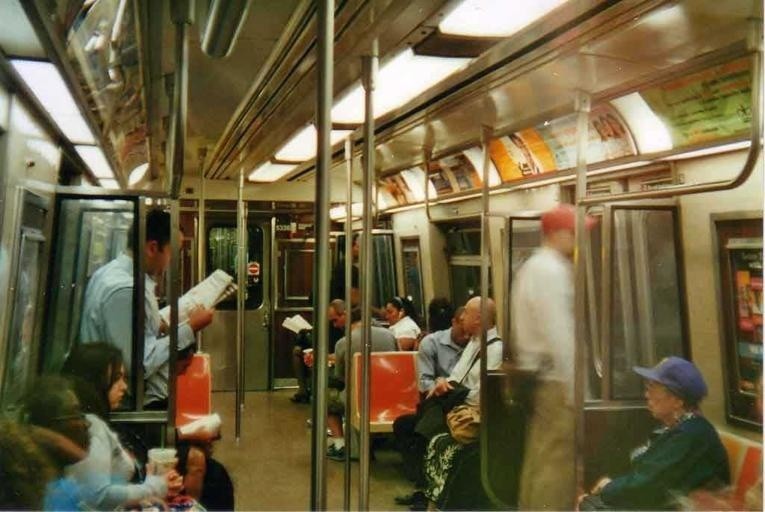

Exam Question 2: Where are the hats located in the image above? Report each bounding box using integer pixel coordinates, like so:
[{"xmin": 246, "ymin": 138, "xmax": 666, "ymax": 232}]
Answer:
[
  {"xmin": 632, "ymin": 356, "xmax": 708, "ymax": 405},
  {"xmin": 541, "ymin": 204, "xmax": 598, "ymax": 236}
]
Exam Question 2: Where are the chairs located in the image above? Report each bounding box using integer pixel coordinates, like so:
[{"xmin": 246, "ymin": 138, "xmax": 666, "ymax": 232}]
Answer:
[{"xmin": 170, "ymin": 351, "xmax": 221, "ymax": 462}]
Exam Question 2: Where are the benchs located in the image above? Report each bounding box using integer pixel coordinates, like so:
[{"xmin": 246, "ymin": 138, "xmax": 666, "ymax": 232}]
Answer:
[
  {"xmin": 667, "ymin": 424, "xmax": 764, "ymax": 510},
  {"xmin": 347, "ymin": 351, "xmax": 427, "ymax": 437}
]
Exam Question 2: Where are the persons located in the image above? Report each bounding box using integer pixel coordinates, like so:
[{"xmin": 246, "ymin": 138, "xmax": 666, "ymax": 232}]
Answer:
[
  {"xmin": 508, "ymin": 133, "xmax": 539, "ymax": 175},
  {"xmin": 593, "ymin": 114, "xmax": 633, "ymax": 160}
]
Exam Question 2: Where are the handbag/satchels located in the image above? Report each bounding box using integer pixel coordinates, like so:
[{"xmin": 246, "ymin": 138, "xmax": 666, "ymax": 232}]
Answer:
[
  {"xmin": 413, "ymin": 381, "xmax": 470, "ymax": 440},
  {"xmin": 159, "ymin": 497, "xmax": 205, "ymax": 511},
  {"xmin": 577, "ymin": 493, "xmax": 622, "ymax": 511},
  {"xmin": 445, "ymin": 403, "xmax": 481, "ymax": 443}
]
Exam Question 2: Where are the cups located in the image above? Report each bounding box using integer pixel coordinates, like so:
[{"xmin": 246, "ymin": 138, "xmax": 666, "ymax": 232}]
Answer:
[
  {"xmin": 302, "ymin": 347, "xmax": 314, "ymax": 368},
  {"xmin": 144, "ymin": 447, "xmax": 181, "ymax": 497}
]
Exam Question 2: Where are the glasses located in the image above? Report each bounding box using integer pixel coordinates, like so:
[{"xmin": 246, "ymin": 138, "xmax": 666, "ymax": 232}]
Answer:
[{"xmin": 47, "ymin": 403, "xmax": 88, "ymax": 424}]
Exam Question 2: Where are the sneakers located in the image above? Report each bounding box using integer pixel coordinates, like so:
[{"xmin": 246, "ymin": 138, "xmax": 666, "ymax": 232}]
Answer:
[{"xmin": 327, "ymin": 444, "xmax": 346, "ymax": 462}]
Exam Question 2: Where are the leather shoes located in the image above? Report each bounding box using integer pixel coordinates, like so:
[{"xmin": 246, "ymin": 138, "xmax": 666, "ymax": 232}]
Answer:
[
  {"xmin": 291, "ymin": 391, "xmax": 310, "ymax": 403},
  {"xmin": 393, "ymin": 490, "xmax": 432, "ymax": 510}
]
